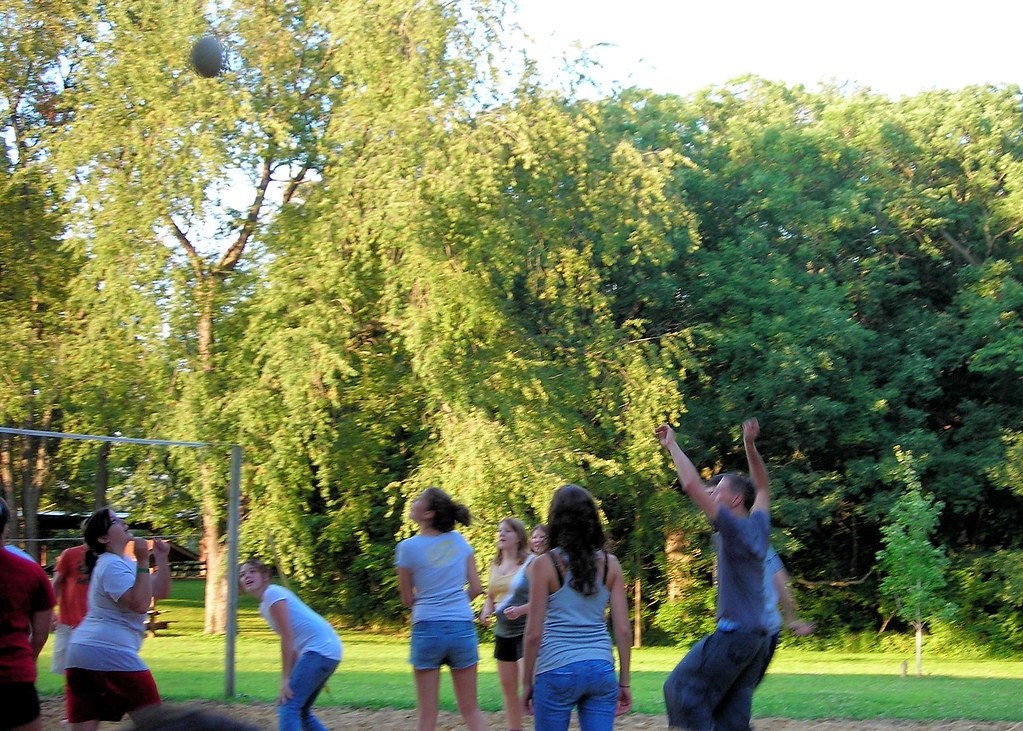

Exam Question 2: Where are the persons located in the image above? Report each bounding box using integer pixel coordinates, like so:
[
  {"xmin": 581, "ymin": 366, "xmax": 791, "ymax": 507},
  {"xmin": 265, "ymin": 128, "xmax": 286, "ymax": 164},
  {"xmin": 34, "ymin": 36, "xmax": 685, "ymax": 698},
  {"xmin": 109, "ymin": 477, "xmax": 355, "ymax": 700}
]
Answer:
[
  {"xmin": 395, "ymin": 487, "xmax": 491, "ymax": 731},
  {"xmin": 481, "ymin": 485, "xmax": 632, "ymax": 731},
  {"xmin": 0, "ymin": 499, "xmax": 56, "ymax": 731},
  {"xmin": 654, "ymin": 418, "xmax": 817, "ymax": 731},
  {"xmin": 53, "ymin": 508, "xmax": 170, "ymax": 731},
  {"xmin": 239, "ymin": 561, "xmax": 344, "ymax": 731}
]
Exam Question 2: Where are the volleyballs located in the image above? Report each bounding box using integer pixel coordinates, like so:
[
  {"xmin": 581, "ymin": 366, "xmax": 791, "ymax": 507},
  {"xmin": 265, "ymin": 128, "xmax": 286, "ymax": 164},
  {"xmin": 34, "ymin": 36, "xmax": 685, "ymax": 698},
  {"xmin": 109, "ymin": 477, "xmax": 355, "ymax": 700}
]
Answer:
[{"xmin": 191, "ymin": 37, "xmax": 222, "ymax": 78}]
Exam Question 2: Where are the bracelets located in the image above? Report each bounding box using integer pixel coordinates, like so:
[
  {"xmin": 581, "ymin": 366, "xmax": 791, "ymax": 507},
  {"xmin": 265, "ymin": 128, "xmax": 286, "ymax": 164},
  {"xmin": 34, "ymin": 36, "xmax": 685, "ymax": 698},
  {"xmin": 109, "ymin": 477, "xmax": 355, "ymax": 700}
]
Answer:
[
  {"xmin": 619, "ymin": 683, "xmax": 630, "ymax": 689},
  {"xmin": 136, "ymin": 567, "xmax": 150, "ymax": 574}
]
